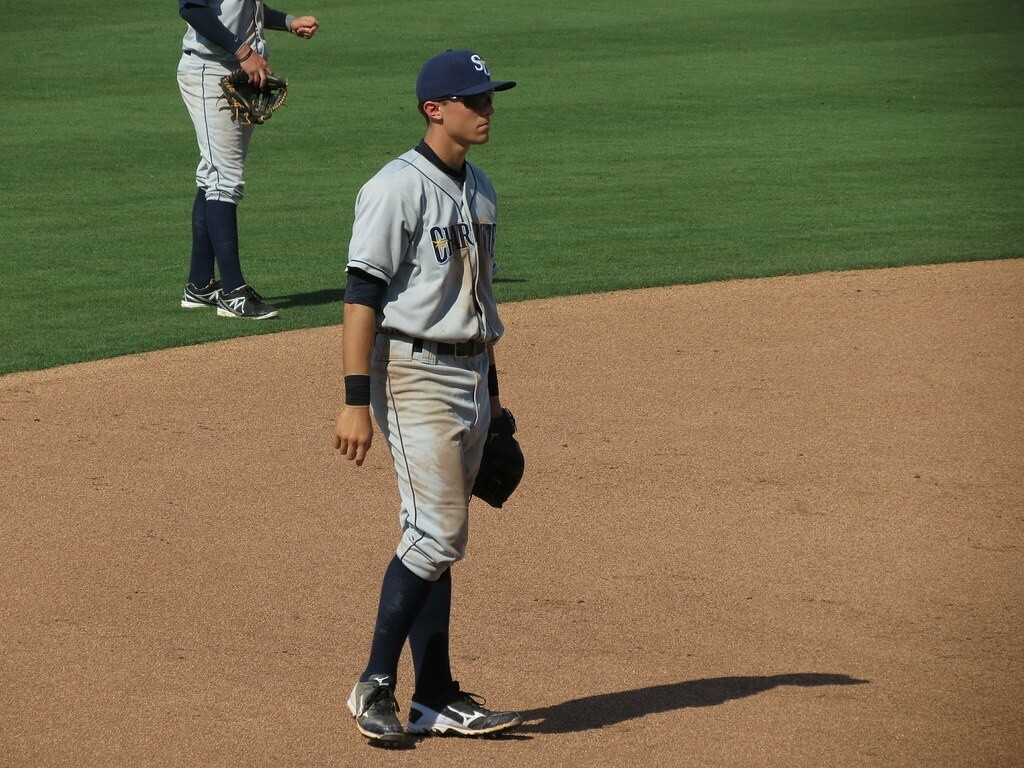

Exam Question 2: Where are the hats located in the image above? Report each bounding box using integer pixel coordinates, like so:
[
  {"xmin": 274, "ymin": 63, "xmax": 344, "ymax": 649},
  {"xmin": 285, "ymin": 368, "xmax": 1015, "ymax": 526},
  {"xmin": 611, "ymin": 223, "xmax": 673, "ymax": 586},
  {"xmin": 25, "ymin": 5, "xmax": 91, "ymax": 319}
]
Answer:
[{"xmin": 416, "ymin": 49, "xmax": 517, "ymax": 106}]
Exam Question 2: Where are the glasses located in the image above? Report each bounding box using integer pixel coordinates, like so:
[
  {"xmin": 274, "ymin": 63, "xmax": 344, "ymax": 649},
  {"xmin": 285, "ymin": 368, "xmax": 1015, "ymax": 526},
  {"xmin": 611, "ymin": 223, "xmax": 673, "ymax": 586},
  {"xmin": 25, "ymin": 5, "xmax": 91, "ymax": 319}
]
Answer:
[{"xmin": 432, "ymin": 89, "xmax": 495, "ymax": 109}]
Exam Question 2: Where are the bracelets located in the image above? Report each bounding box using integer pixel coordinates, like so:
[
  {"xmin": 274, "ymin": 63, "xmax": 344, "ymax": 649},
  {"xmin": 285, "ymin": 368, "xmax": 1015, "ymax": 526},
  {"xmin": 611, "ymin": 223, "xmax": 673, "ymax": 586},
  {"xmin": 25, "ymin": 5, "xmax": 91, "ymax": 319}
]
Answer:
[
  {"xmin": 344, "ymin": 374, "xmax": 370, "ymax": 406},
  {"xmin": 237, "ymin": 47, "xmax": 253, "ymax": 62}
]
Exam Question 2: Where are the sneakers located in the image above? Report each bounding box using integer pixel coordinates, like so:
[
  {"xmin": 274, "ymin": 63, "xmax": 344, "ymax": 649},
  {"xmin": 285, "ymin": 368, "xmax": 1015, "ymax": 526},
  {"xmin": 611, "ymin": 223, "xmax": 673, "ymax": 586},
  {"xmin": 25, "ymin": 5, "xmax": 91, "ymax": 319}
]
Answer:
[
  {"xmin": 180, "ymin": 277, "xmax": 224, "ymax": 310},
  {"xmin": 218, "ymin": 284, "xmax": 278, "ymax": 320},
  {"xmin": 346, "ymin": 671, "xmax": 404, "ymax": 741},
  {"xmin": 404, "ymin": 681, "xmax": 522, "ymax": 736}
]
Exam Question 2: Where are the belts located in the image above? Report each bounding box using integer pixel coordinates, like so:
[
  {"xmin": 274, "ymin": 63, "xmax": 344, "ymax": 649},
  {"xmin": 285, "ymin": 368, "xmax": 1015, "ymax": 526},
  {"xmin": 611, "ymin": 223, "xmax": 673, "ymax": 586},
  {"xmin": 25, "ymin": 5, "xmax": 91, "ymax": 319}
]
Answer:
[
  {"xmin": 374, "ymin": 332, "xmax": 485, "ymax": 358},
  {"xmin": 183, "ymin": 49, "xmax": 191, "ymax": 56}
]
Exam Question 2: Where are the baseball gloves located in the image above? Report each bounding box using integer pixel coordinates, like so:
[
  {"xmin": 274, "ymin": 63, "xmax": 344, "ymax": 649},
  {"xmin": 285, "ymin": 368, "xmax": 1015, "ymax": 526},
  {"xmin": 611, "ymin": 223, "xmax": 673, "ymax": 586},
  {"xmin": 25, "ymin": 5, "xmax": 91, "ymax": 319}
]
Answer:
[
  {"xmin": 471, "ymin": 407, "xmax": 525, "ymax": 510},
  {"xmin": 220, "ymin": 69, "xmax": 287, "ymax": 127}
]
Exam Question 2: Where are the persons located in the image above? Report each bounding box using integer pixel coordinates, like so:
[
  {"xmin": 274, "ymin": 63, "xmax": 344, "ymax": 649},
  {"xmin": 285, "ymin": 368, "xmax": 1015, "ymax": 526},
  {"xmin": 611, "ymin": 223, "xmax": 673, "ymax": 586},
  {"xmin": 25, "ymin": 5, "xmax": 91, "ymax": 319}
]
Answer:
[
  {"xmin": 176, "ymin": 0, "xmax": 319, "ymax": 320},
  {"xmin": 334, "ymin": 50, "xmax": 524, "ymax": 740}
]
